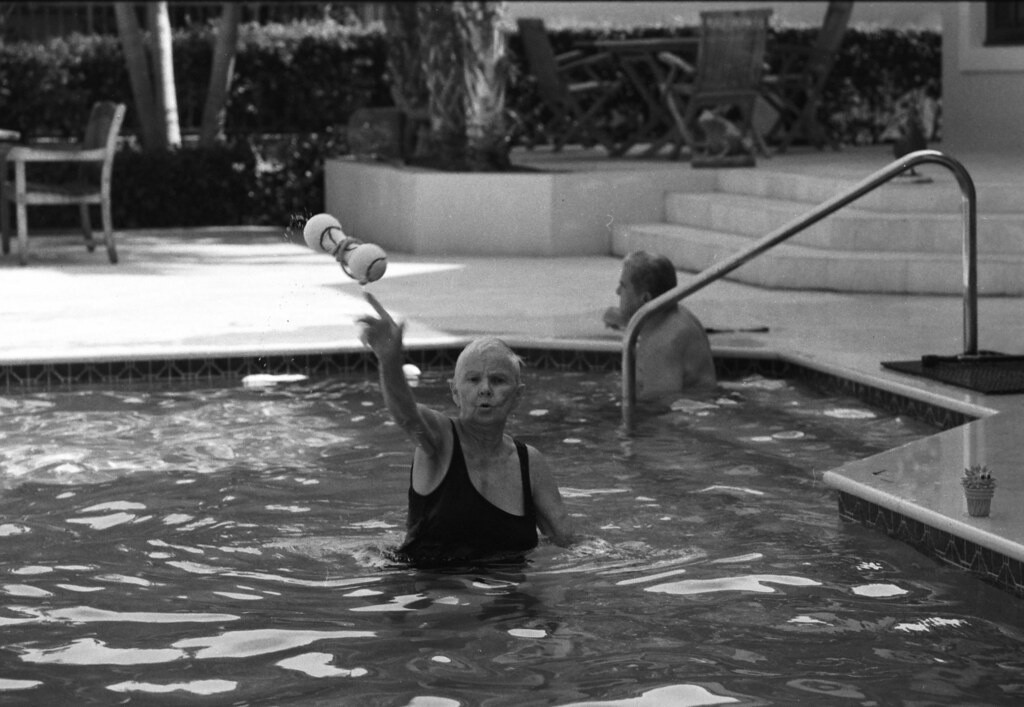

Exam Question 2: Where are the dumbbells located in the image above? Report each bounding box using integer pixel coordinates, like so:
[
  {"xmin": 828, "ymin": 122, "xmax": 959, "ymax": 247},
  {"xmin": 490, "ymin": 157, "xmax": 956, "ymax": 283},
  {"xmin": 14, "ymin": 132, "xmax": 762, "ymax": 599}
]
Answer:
[{"xmin": 304, "ymin": 213, "xmax": 388, "ymax": 284}]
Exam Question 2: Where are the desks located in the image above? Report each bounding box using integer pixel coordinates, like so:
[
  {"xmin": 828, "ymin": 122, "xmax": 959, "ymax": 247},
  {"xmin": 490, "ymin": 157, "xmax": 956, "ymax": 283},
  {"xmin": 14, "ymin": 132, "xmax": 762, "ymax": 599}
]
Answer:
[
  {"xmin": 573, "ymin": 32, "xmax": 776, "ymax": 159},
  {"xmin": 668, "ymin": 130, "xmax": 675, "ymax": 132}
]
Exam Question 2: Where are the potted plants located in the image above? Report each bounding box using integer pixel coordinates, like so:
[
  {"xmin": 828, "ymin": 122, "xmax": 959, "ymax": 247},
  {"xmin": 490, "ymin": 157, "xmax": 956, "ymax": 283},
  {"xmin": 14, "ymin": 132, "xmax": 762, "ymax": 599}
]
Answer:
[{"xmin": 960, "ymin": 463, "xmax": 996, "ymax": 517}]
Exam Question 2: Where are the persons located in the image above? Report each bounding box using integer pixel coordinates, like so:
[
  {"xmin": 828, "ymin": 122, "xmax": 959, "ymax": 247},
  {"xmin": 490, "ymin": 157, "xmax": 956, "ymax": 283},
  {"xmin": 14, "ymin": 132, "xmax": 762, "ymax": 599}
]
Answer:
[
  {"xmin": 352, "ymin": 292, "xmax": 582, "ymax": 562},
  {"xmin": 604, "ymin": 249, "xmax": 718, "ymax": 396}
]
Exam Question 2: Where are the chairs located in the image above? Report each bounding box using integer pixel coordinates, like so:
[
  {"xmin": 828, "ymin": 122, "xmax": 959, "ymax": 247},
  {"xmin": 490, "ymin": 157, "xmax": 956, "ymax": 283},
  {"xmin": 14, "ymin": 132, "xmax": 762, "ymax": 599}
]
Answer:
[
  {"xmin": 1, "ymin": 100, "xmax": 126, "ymax": 267},
  {"xmin": 506, "ymin": 17, "xmax": 625, "ymax": 158},
  {"xmin": 648, "ymin": 6, "xmax": 774, "ymax": 168},
  {"xmin": 755, "ymin": 0, "xmax": 855, "ymax": 153}
]
